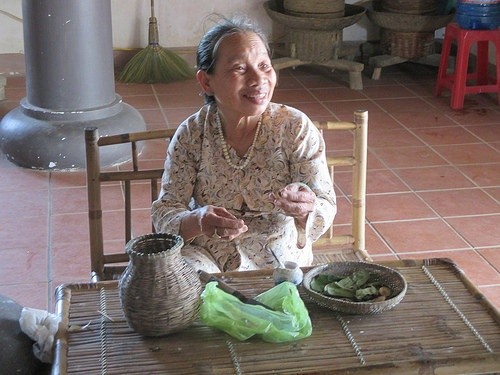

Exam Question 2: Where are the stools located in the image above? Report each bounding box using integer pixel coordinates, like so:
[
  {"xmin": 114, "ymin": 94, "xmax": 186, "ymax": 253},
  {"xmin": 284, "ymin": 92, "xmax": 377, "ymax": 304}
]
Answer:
[{"xmin": 433, "ymin": 23, "xmax": 500, "ymax": 111}]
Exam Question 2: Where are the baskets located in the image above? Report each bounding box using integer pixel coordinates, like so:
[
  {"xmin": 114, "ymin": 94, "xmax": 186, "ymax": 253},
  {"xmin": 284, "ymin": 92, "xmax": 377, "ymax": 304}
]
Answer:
[
  {"xmin": 119, "ymin": 233, "xmax": 202, "ymax": 336},
  {"xmin": 303, "ymin": 261, "xmax": 407, "ymax": 314},
  {"xmin": 263, "ymin": 0, "xmax": 367, "ymax": 61},
  {"xmin": 365, "ymin": 0, "xmax": 456, "ymax": 58}
]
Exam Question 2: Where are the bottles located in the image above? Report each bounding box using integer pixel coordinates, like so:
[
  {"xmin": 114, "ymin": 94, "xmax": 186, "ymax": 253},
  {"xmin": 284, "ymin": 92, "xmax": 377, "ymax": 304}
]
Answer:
[{"xmin": 457, "ymin": 0, "xmax": 500, "ymax": 31}]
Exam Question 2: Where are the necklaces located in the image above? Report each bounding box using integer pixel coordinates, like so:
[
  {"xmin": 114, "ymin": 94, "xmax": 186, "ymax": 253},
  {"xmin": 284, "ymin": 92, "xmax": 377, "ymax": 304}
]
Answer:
[{"xmin": 216, "ymin": 108, "xmax": 262, "ymax": 170}]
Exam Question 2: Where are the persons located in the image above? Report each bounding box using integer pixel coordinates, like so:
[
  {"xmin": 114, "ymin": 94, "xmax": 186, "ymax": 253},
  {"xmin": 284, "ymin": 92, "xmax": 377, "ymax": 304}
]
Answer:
[{"xmin": 150, "ymin": 19, "xmax": 339, "ymax": 273}]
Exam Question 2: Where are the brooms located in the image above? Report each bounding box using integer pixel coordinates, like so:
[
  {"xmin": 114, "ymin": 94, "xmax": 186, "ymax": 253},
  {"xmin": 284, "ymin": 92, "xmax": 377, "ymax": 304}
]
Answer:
[{"xmin": 114, "ymin": 0, "xmax": 195, "ymax": 86}]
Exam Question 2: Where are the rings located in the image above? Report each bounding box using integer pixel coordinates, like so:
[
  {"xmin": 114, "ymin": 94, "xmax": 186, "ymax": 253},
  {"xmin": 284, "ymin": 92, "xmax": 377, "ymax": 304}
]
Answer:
[{"xmin": 211, "ymin": 226, "xmax": 221, "ymax": 240}]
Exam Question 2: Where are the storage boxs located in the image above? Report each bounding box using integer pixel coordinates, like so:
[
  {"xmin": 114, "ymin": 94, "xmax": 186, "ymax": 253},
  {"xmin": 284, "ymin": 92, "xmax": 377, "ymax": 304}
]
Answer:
[
  {"xmin": 263, "ymin": 0, "xmax": 367, "ymax": 61},
  {"xmin": 366, "ymin": 0, "xmax": 457, "ymax": 60}
]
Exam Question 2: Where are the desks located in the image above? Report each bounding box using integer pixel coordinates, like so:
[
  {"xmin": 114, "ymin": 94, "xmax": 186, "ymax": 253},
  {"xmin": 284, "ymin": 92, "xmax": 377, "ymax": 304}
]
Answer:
[{"xmin": 51, "ymin": 256, "xmax": 500, "ymax": 375}]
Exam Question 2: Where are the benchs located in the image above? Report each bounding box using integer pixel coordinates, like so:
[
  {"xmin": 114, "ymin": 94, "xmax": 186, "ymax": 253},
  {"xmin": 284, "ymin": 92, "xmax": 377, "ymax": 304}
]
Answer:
[{"xmin": 85, "ymin": 109, "xmax": 372, "ymax": 283}]
